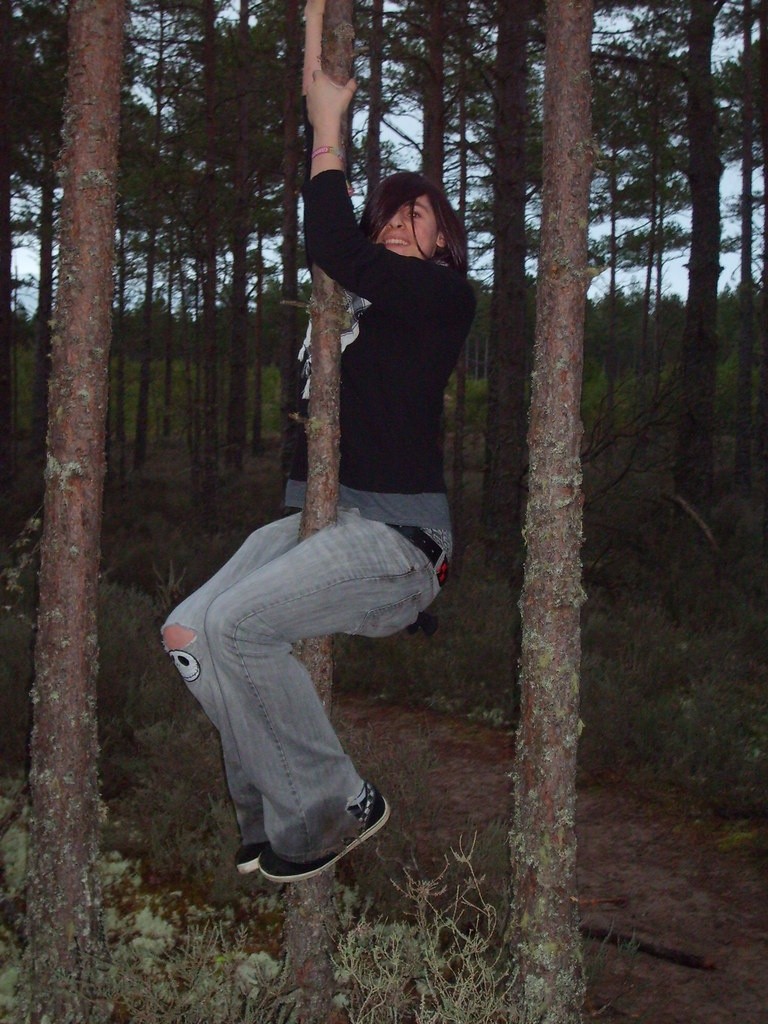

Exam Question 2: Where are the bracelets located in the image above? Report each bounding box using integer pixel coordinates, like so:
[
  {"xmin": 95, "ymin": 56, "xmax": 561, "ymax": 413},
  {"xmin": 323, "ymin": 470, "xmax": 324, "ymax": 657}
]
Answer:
[{"xmin": 311, "ymin": 147, "xmax": 354, "ymax": 195}]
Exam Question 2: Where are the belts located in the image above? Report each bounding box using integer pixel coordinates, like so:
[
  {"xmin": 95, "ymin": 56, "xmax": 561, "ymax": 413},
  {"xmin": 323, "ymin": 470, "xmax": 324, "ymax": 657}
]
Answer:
[{"xmin": 386, "ymin": 521, "xmax": 451, "ymax": 588}]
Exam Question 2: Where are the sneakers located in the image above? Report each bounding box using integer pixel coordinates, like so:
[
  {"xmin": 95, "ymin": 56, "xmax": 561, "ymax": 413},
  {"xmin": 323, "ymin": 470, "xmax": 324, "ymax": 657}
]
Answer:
[
  {"xmin": 235, "ymin": 841, "xmax": 269, "ymax": 874},
  {"xmin": 258, "ymin": 781, "xmax": 390, "ymax": 883}
]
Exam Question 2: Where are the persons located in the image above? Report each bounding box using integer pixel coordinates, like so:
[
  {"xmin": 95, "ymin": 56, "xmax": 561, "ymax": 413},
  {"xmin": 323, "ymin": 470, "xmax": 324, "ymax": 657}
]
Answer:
[{"xmin": 159, "ymin": 1, "xmax": 471, "ymax": 884}]
